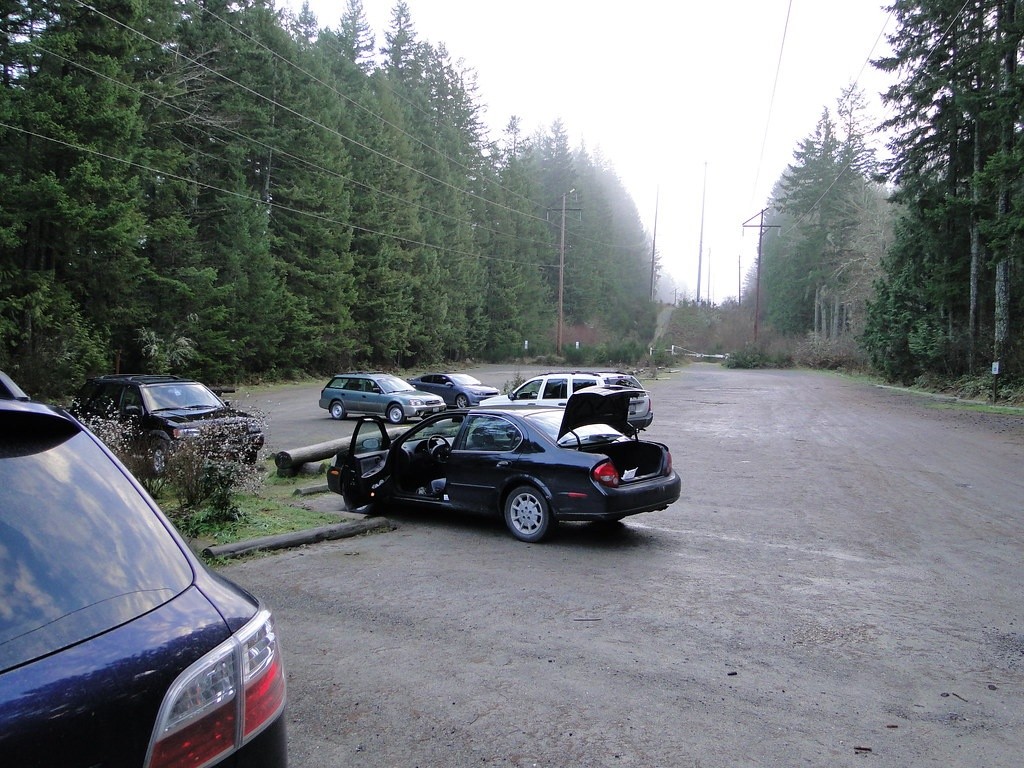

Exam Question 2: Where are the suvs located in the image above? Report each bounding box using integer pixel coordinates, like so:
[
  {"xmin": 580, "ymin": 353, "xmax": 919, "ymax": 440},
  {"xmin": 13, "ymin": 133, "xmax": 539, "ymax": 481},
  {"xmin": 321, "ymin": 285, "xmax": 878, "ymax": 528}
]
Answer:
[
  {"xmin": 478, "ymin": 370, "xmax": 653, "ymax": 439},
  {"xmin": 69, "ymin": 373, "xmax": 264, "ymax": 482},
  {"xmin": 318, "ymin": 370, "xmax": 446, "ymax": 425}
]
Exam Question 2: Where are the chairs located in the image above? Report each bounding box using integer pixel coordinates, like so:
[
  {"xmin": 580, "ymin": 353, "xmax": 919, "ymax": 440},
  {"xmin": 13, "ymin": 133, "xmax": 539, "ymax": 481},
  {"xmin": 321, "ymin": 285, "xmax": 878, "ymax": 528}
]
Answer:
[{"xmin": 471, "ymin": 426, "xmax": 502, "ymax": 450}]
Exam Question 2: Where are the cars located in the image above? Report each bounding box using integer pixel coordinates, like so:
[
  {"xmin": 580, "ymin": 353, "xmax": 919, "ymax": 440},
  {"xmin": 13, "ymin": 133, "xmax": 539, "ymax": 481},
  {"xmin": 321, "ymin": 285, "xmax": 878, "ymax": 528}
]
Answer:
[
  {"xmin": 325, "ymin": 383, "xmax": 685, "ymax": 543},
  {"xmin": 1, "ymin": 396, "xmax": 289, "ymax": 768},
  {"xmin": 406, "ymin": 373, "xmax": 501, "ymax": 410}
]
